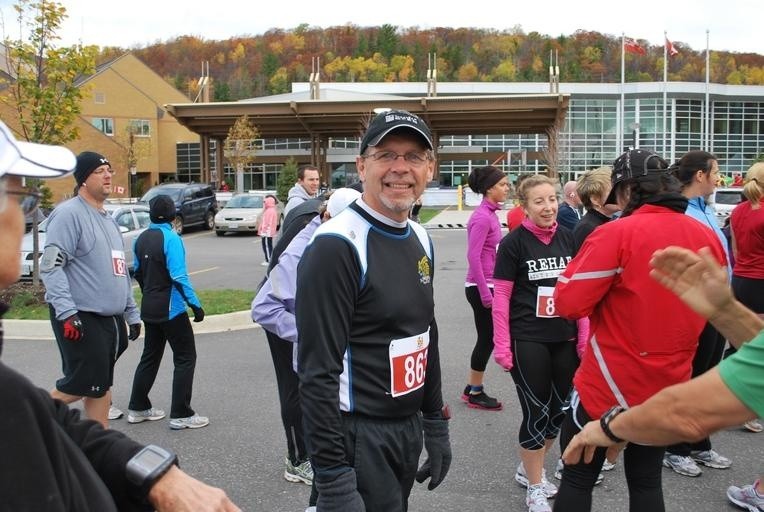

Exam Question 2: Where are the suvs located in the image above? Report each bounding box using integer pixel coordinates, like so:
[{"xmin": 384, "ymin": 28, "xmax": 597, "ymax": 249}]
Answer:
[{"xmin": 137, "ymin": 184, "xmax": 217, "ymax": 235}]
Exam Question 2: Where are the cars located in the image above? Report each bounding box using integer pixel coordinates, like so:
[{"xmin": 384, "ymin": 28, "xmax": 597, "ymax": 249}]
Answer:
[
  {"xmin": 20, "ymin": 202, "xmax": 159, "ymax": 280},
  {"xmin": 705, "ymin": 188, "xmax": 747, "ymax": 229},
  {"xmin": 213, "ymin": 192, "xmax": 284, "ymax": 236}
]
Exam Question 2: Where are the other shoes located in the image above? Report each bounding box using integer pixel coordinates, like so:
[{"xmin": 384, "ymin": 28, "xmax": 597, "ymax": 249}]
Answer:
[{"xmin": 744, "ymin": 419, "xmax": 763, "ymax": 432}]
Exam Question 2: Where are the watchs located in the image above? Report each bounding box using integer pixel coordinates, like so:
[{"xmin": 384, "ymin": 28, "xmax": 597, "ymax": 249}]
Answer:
[{"xmin": 124, "ymin": 442, "xmax": 179, "ymax": 511}]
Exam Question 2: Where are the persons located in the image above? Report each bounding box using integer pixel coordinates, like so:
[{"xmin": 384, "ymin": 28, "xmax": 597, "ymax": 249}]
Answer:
[
  {"xmin": 39, "ymin": 150, "xmax": 142, "ymax": 431},
  {"xmin": 0, "ymin": 119, "xmax": 242, "ymax": 512},
  {"xmin": 461, "ymin": 166, "xmax": 504, "ymax": 411},
  {"xmin": 293, "ymin": 108, "xmax": 452, "ymax": 512},
  {"xmin": 126, "ymin": 194, "xmax": 210, "ymax": 430},
  {"xmin": 20, "ymin": 183, "xmax": 47, "ymax": 234},
  {"xmin": 250, "ymin": 164, "xmax": 361, "ymax": 487},
  {"xmin": 550, "ymin": 147, "xmax": 763, "ymax": 510},
  {"xmin": 492, "ymin": 174, "xmax": 589, "ymax": 512}
]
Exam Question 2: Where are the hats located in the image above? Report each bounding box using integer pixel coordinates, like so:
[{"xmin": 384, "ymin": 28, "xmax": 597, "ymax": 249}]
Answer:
[
  {"xmin": 149, "ymin": 195, "xmax": 176, "ymax": 224},
  {"xmin": 360, "ymin": 109, "xmax": 434, "ymax": 154},
  {"xmin": 603, "ymin": 149, "xmax": 680, "ymax": 207},
  {"xmin": 74, "ymin": 151, "xmax": 111, "ymax": 187},
  {"xmin": 0, "ymin": 120, "xmax": 77, "ymax": 179},
  {"xmin": 327, "ymin": 187, "xmax": 361, "ymax": 217}
]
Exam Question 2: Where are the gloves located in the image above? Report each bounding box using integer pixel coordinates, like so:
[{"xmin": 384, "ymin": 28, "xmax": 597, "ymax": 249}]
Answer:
[
  {"xmin": 193, "ymin": 308, "xmax": 204, "ymax": 322},
  {"xmin": 128, "ymin": 324, "xmax": 140, "ymax": 341},
  {"xmin": 417, "ymin": 418, "xmax": 452, "ymax": 490},
  {"xmin": 64, "ymin": 315, "xmax": 85, "ymax": 342},
  {"xmin": 314, "ymin": 467, "xmax": 365, "ymax": 511}
]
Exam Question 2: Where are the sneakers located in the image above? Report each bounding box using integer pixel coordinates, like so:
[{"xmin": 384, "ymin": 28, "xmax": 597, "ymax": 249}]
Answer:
[
  {"xmin": 726, "ymin": 480, "xmax": 763, "ymax": 512},
  {"xmin": 108, "ymin": 405, "xmax": 208, "ymax": 429},
  {"xmin": 661, "ymin": 451, "xmax": 702, "ymax": 476},
  {"xmin": 555, "ymin": 458, "xmax": 604, "ymax": 485},
  {"xmin": 601, "ymin": 459, "xmax": 615, "ymax": 471},
  {"xmin": 690, "ymin": 450, "xmax": 732, "ymax": 469},
  {"xmin": 515, "ymin": 461, "xmax": 559, "ymax": 498},
  {"xmin": 525, "ymin": 481, "xmax": 553, "ymax": 512},
  {"xmin": 284, "ymin": 460, "xmax": 312, "ymax": 485},
  {"xmin": 463, "ymin": 386, "xmax": 503, "ymax": 411}
]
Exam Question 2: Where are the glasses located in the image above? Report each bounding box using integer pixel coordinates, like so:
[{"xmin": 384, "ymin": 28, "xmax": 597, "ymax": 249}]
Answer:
[
  {"xmin": 362, "ymin": 150, "xmax": 429, "ymax": 164},
  {"xmin": 94, "ymin": 169, "xmax": 116, "ymax": 175},
  {"xmin": 6, "ymin": 184, "xmax": 40, "ymax": 217}
]
